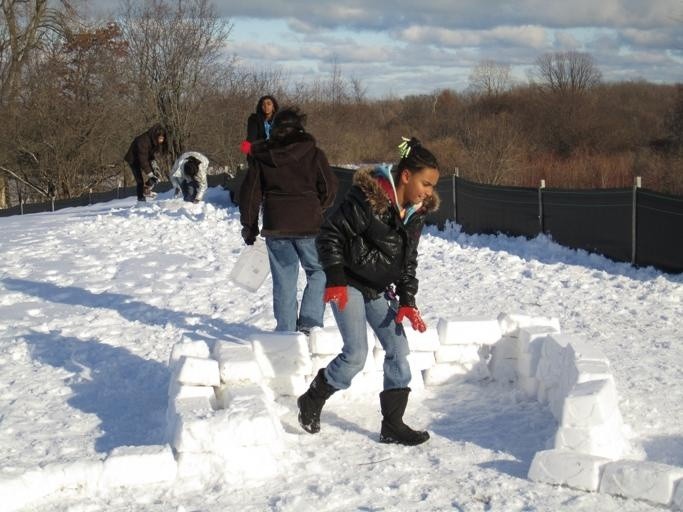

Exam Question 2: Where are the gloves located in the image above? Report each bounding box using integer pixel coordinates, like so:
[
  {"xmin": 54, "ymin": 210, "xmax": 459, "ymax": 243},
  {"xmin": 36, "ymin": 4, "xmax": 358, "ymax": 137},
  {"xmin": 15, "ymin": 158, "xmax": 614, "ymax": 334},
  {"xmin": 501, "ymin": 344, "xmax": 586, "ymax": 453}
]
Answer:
[
  {"xmin": 242, "ymin": 228, "xmax": 259, "ymax": 244},
  {"xmin": 325, "ymin": 286, "xmax": 347, "ymax": 309},
  {"xmin": 396, "ymin": 307, "xmax": 426, "ymax": 332}
]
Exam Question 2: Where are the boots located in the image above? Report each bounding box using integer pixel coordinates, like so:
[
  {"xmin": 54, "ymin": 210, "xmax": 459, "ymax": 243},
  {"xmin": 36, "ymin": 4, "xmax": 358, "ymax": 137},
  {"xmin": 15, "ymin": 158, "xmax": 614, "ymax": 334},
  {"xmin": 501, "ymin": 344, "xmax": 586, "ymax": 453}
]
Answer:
[
  {"xmin": 298, "ymin": 368, "xmax": 340, "ymax": 433},
  {"xmin": 380, "ymin": 388, "xmax": 429, "ymax": 445}
]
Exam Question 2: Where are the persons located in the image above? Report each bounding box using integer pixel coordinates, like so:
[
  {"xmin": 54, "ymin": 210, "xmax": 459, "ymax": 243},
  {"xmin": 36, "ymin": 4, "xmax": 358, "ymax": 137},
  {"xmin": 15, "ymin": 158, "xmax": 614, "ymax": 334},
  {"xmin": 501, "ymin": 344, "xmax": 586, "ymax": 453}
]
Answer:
[
  {"xmin": 170, "ymin": 150, "xmax": 210, "ymax": 204},
  {"xmin": 246, "ymin": 95, "xmax": 279, "ymax": 168},
  {"xmin": 297, "ymin": 137, "xmax": 441, "ymax": 446},
  {"xmin": 240, "ymin": 105, "xmax": 340, "ymax": 336},
  {"xmin": 124, "ymin": 123, "xmax": 168, "ymax": 202}
]
